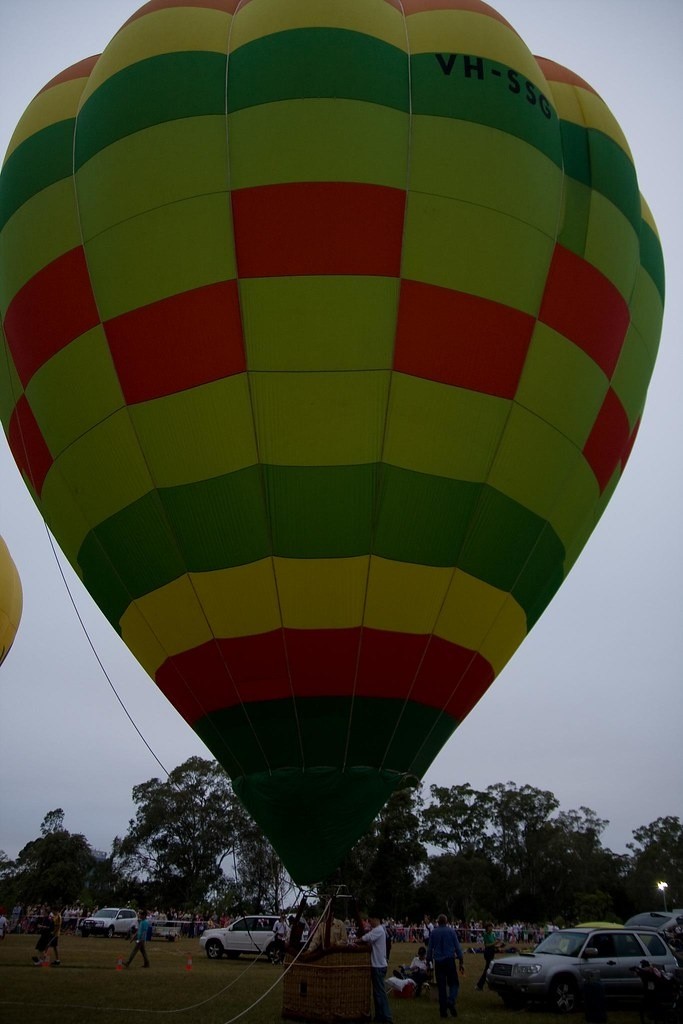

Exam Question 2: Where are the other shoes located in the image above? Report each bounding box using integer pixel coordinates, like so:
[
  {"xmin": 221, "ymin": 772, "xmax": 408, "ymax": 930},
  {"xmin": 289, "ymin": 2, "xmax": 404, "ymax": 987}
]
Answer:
[
  {"xmin": 448, "ymin": 1003, "xmax": 458, "ymax": 1017},
  {"xmin": 393, "ymin": 970, "xmax": 404, "ymax": 981},
  {"xmin": 49, "ymin": 961, "xmax": 61, "ymax": 967},
  {"xmin": 473, "ymin": 985, "xmax": 481, "ymax": 992},
  {"xmin": 32, "ymin": 958, "xmax": 40, "ymax": 965}
]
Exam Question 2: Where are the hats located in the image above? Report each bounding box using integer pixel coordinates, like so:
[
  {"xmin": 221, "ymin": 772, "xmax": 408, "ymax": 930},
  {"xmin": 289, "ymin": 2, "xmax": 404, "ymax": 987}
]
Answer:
[{"xmin": 138, "ymin": 910, "xmax": 148, "ymax": 915}]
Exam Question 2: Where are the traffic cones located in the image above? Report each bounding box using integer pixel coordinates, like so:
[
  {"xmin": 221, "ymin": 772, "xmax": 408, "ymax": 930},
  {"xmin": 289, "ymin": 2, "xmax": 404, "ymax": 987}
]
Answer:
[
  {"xmin": 40, "ymin": 949, "xmax": 50, "ymax": 967},
  {"xmin": 116, "ymin": 954, "xmax": 124, "ymax": 971},
  {"xmin": 185, "ymin": 955, "xmax": 193, "ymax": 970}
]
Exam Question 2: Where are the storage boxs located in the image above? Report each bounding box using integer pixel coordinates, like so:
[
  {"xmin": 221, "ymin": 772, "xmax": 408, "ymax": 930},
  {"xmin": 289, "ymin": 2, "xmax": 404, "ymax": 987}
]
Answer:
[{"xmin": 390, "ymin": 983, "xmax": 412, "ymax": 997}]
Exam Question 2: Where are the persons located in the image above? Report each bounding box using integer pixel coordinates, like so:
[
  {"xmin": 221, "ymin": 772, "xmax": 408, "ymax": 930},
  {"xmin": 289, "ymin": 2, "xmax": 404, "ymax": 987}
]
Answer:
[
  {"xmin": 425, "ymin": 914, "xmax": 464, "ymax": 1019},
  {"xmin": 398, "ymin": 946, "xmax": 434, "ymax": 1000},
  {"xmin": 121, "ymin": 909, "xmax": 150, "ymax": 969},
  {"xmin": 299, "ymin": 906, "xmax": 349, "ymax": 958},
  {"xmin": 0, "ymin": 896, "xmax": 568, "ymax": 966},
  {"xmin": 271, "ymin": 912, "xmax": 289, "ymax": 965},
  {"xmin": 354, "ymin": 911, "xmax": 395, "ymax": 1024},
  {"xmin": 475, "ymin": 923, "xmax": 504, "ymax": 992},
  {"xmin": 664, "ymin": 915, "xmax": 683, "ymax": 943}
]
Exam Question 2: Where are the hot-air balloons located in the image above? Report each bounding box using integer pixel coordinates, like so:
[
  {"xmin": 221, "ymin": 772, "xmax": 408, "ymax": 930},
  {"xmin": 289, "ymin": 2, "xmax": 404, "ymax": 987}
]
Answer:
[{"xmin": 0, "ymin": 0, "xmax": 668, "ymax": 1024}]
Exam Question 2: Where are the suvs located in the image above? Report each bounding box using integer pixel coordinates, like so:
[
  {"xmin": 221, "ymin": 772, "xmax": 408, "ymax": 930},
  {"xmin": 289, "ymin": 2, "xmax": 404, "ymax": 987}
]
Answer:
[
  {"xmin": 485, "ymin": 912, "xmax": 680, "ymax": 1012},
  {"xmin": 200, "ymin": 915, "xmax": 308, "ymax": 962},
  {"xmin": 80, "ymin": 906, "xmax": 139, "ymax": 938}
]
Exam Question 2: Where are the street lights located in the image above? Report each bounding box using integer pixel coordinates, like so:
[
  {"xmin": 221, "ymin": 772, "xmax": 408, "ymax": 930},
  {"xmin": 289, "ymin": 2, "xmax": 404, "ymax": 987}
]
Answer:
[{"xmin": 657, "ymin": 881, "xmax": 668, "ymax": 912}]
